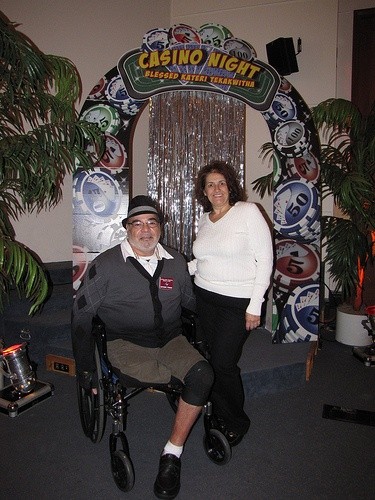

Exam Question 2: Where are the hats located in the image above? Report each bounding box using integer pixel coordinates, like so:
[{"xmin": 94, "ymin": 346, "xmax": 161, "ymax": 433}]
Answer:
[{"xmin": 121, "ymin": 195, "xmax": 165, "ymax": 230}]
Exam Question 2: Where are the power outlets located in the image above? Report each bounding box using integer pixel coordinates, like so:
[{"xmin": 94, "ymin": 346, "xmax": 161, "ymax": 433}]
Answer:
[{"xmin": 54, "ymin": 361, "xmax": 69, "ymax": 372}]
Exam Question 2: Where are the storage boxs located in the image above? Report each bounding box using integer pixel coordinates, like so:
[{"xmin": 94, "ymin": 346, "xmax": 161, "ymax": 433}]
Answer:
[{"xmin": 0, "ymin": 380, "xmax": 56, "ymax": 418}]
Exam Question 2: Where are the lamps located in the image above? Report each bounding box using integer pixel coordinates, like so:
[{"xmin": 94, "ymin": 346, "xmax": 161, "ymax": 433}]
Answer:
[{"xmin": 0, "ymin": 342, "xmax": 38, "ymax": 394}]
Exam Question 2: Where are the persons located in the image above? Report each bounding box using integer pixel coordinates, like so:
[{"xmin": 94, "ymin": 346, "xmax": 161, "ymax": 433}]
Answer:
[
  {"xmin": 70, "ymin": 197, "xmax": 213, "ymax": 500},
  {"xmin": 186, "ymin": 161, "xmax": 273, "ymax": 431}
]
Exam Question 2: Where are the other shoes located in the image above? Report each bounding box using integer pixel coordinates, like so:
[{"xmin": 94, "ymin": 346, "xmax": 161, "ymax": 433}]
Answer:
[{"xmin": 214, "ymin": 418, "xmax": 250, "ymax": 448}]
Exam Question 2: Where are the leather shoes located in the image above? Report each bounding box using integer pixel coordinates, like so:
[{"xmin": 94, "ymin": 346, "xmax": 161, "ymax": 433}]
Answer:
[{"xmin": 153, "ymin": 449, "xmax": 182, "ymax": 500}]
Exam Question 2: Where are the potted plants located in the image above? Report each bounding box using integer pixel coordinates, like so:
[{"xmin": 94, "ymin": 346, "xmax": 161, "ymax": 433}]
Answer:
[{"xmin": 250, "ymin": 99, "xmax": 375, "ymax": 347}]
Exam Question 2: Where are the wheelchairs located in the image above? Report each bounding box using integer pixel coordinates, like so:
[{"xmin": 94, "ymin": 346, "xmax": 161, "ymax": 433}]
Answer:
[{"xmin": 73, "ymin": 308, "xmax": 233, "ymax": 494}]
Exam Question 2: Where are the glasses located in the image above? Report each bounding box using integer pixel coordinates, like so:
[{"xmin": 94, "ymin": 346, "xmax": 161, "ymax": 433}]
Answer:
[{"xmin": 126, "ymin": 219, "xmax": 162, "ymax": 228}]
